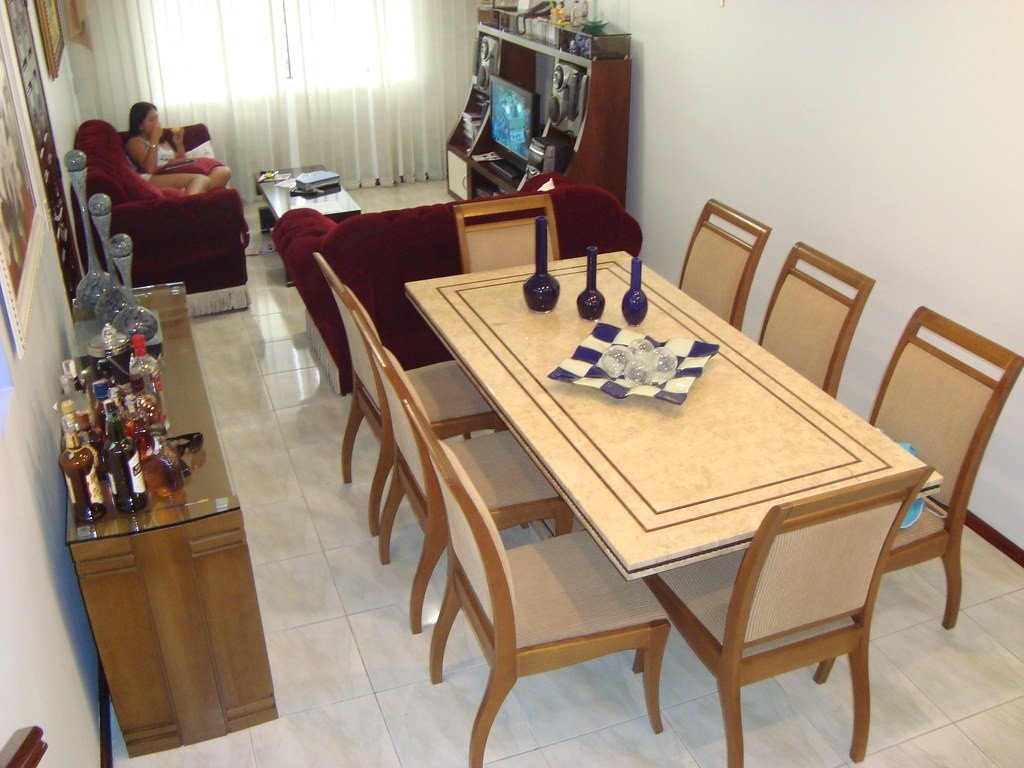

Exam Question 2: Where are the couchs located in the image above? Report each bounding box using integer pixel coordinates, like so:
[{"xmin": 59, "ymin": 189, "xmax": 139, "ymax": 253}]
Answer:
[
  {"xmin": 268, "ymin": 172, "xmax": 642, "ymax": 397},
  {"xmin": 70, "ymin": 120, "xmax": 258, "ymax": 314}
]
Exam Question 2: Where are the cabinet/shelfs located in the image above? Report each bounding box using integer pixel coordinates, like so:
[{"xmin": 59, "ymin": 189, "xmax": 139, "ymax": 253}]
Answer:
[
  {"xmin": 64, "ymin": 281, "xmax": 279, "ymax": 759},
  {"xmin": 445, "ymin": 0, "xmax": 632, "ymax": 210}
]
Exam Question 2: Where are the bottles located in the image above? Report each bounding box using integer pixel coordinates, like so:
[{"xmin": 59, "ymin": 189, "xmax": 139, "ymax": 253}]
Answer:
[
  {"xmin": 571, "ymin": 0, "xmax": 583, "ymax": 26},
  {"xmin": 621, "ymin": 259, "xmax": 648, "ymax": 327},
  {"xmin": 523, "ymin": 216, "xmax": 559, "ymax": 314},
  {"xmin": 54, "ymin": 325, "xmax": 184, "ymax": 523},
  {"xmin": 577, "ymin": 245, "xmax": 605, "ymax": 320}
]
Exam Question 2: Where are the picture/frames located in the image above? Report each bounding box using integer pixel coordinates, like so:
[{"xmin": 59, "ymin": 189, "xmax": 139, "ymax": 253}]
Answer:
[
  {"xmin": 0, "ymin": 13, "xmax": 46, "ymax": 359},
  {"xmin": 34, "ymin": 0, "xmax": 65, "ymax": 81}
]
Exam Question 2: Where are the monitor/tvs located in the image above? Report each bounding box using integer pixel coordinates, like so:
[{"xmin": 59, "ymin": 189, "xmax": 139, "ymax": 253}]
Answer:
[{"xmin": 489, "ymin": 75, "xmax": 541, "ymax": 180}]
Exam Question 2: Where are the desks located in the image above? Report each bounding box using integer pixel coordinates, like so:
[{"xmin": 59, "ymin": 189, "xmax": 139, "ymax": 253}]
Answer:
[{"xmin": 404, "ymin": 251, "xmax": 946, "ymax": 583}]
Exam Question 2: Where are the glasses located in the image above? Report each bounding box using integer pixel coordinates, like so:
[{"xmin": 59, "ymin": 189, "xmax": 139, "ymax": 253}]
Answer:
[{"xmin": 166, "ymin": 432, "xmax": 205, "ymax": 457}]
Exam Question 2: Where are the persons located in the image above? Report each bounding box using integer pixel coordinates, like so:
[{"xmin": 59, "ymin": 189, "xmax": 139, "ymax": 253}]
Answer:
[
  {"xmin": 550, "ymin": 1, "xmax": 588, "ymax": 27},
  {"xmin": 126, "ymin": 102, "xmax": 232, "ymax": 195}
]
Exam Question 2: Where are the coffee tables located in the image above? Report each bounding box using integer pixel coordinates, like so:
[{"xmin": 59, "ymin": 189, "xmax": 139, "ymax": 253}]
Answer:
[{"xmin": 251, "ymin": 164, "xmax": 362, "ymax": 287}]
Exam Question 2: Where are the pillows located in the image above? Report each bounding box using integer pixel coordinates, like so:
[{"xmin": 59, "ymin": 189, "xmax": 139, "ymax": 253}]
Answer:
[{"xmin": 153, "ymin": 157, "xmax": 226, "ymax": 175}]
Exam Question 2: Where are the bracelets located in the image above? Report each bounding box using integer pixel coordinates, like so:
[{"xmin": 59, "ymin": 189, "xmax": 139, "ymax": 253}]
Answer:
[{"xmin": 149, "ymin": 143, "xmax": 158, "ymax": 150}]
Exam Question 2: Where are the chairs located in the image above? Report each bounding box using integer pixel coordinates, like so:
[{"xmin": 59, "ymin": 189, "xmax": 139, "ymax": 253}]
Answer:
[
  {"xmin": 452, "ymin": 194, "xmax": 561, "ymax": 274},
  {"xmin": 403, "ymin": 398, "xmax": 671, "ymax": 768},
  {"xmin": 814, "ymin": 305, "xmax": 1024, "ymax": 685},
  {"xmin": 313, "ymin": 251, "xmax": 531, "ymax": 538},
  {"xmin": 351, "ymin": 307, "xmax": 574, "ymax": 635},
  {"xmin": 677, "ymin": 199, "xmax": 772, "ymax": 333},
  {"xmin": 631, "ymin": 465, "xmax": 938, "ymax": 768},
  {"xmin": 758, "ymin": 242, "xmax": 877, "ymax": 400}
]
